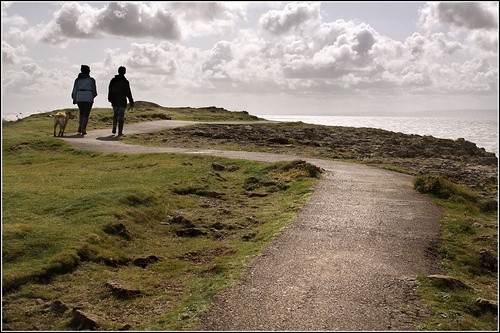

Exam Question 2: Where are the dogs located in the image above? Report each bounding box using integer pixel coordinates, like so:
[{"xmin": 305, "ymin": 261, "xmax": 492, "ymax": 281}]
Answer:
[{"xmin": 49, "ymin": 111, "xmax": 74, "ymax": 137}]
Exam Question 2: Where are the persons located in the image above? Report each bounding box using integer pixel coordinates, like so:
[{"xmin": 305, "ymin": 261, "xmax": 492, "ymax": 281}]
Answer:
[
  {"xmin": 71, "ymin": 65, "xmax": 97, "ymax": 135},
  {"xmin": 108, "ymin": 66, "xmax": 135, "ymax": 136}
]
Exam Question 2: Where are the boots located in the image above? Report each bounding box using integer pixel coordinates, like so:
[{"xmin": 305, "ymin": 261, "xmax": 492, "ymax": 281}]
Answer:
[{"xmin": 78, "ymin": 116, "xmax": 87, "ymax": 135}]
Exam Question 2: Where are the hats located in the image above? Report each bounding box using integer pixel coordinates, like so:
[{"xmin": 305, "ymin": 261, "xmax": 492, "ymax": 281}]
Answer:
[{"xmin": 80, "ymin": 64, "xmax": 89, "ymax": 72}]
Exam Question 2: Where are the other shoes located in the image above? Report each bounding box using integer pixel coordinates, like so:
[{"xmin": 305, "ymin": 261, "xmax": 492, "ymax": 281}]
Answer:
[
  {"xmin": 112, "ymin": 130, "xmax": 116, "ymax": 133},
  {"xmin": 119, "ymin": 133, "xmax": 124, "ymax": 136}
]
